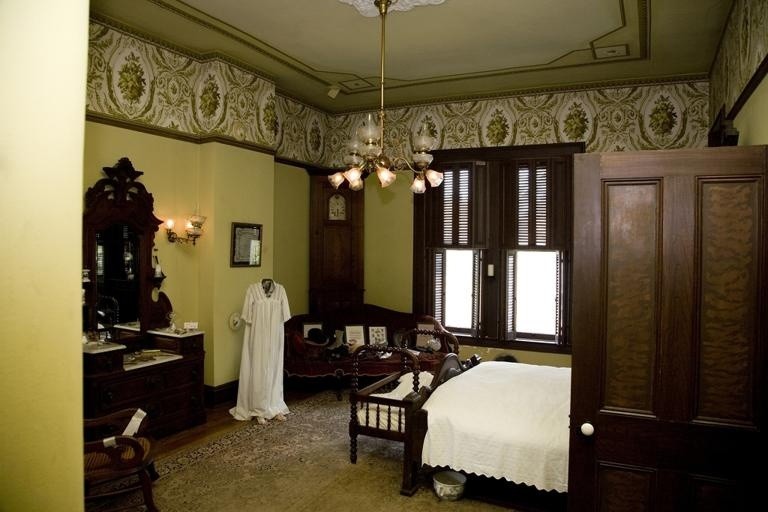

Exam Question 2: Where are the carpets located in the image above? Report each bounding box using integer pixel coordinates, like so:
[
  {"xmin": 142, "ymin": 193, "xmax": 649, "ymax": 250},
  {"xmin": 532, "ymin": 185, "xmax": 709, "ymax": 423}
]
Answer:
[{"xmin": 146, "ymin": 385, "xmax": 567, "ymax": 512}]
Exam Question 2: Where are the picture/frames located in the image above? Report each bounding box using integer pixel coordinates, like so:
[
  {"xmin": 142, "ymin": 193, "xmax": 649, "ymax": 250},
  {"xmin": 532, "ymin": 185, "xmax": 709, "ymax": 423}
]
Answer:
[{"xmin": 230, "ymin": 222, "xmax": 263, "ymax": 267}]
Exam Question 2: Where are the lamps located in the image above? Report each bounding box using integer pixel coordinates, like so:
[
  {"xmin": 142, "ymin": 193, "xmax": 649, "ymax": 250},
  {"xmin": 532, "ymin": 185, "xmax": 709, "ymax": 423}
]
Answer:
[
  {"xmin": 167, "ymin": 214, "xmax": 207, "ymax": 246},
  {"xmin": 328, "ymin": 1, "xmax": 444, "ymax": 194}
]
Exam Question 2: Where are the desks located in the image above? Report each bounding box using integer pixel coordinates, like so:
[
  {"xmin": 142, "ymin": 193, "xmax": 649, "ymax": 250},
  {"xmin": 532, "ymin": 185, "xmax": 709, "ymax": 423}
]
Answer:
[{"xmin": 84, "ymin": 320, "xmax": 209, "ymax": 450}]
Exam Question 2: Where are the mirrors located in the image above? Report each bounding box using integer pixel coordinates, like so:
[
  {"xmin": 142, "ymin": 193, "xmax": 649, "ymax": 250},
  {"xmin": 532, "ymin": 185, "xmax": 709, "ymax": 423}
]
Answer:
[{"xmin": 81, "ymin": 158, "xmax": 172, "ymax": 343}]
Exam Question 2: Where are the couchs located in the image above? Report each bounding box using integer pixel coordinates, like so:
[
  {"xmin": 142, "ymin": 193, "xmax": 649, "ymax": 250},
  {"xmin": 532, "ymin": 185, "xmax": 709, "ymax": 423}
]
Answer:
[{"xmin": 284, "ymin": 305, "xmax": 450, "ymax": 401}]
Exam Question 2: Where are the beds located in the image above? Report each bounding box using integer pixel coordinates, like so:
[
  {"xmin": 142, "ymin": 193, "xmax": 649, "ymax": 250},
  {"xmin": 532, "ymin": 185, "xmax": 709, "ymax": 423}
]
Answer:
[
  {"xmin": 349, "ymin": 330, "xmax": 459, "ymax": 481},
  {"xmin": 403, "ymin": 353, "xmax": 572, "ymax": 512}
]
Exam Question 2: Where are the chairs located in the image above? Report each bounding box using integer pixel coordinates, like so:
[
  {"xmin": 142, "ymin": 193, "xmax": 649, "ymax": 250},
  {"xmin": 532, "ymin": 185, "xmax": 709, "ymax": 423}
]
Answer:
[{"xmin": 84, "ymin": 407, "xmax": 161, "ymax": 512}]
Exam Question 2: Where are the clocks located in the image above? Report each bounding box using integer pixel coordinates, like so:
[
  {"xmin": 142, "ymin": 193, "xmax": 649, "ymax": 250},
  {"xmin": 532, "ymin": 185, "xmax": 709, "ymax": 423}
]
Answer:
[{"xmin": 329, "ymin": 191, "xmax": 346, "ymax": 220}]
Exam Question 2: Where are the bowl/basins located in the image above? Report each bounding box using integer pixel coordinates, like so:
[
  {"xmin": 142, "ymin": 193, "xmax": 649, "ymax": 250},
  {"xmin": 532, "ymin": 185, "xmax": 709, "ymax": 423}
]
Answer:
[{"xmin": 432, "ymin": 470, "xmax": 467, "ymax": 501}]
